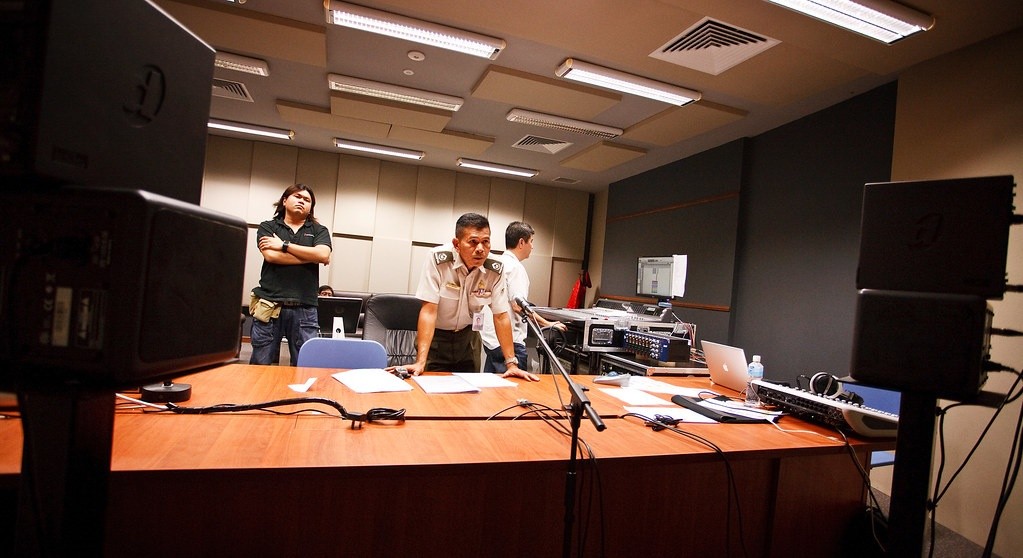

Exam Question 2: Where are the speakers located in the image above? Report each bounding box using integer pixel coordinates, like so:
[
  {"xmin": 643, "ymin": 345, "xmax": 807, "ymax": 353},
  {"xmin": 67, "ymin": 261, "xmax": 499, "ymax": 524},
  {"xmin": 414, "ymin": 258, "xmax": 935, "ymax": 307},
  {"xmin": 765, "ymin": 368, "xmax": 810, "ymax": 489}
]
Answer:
[
  {"xmin": 851, "ymin": 175, "xmax": 1014, "ymax": 393},
  {"xmin": 0, "ymin": 0, "xmax": 247, "ymax": 395}
]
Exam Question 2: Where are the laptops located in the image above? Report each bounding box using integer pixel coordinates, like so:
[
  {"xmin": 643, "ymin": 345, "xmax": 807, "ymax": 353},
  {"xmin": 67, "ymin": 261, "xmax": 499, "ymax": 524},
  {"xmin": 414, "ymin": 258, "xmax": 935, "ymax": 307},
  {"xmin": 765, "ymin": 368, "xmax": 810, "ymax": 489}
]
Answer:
[{"xmin": 701, "ymin": 340, "xmax": 750, "ymax": 395}]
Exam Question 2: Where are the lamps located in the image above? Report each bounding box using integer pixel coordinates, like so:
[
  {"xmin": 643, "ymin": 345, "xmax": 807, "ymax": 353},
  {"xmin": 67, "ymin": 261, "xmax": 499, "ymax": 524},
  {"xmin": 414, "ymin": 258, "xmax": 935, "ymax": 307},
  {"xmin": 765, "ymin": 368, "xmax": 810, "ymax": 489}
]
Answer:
[
  {"xmin": 323, "ymin": 0, "xmax": 505, "ymax": 62},
  {"xmin": 457, "ymin": 158, "xmax": 539, "ymax": 179},
  {"xmin": 505, "ymin": 108, "xmax": 624, "ymax": 141},
  {"xmin": 207, "ymin": 118, "xmax": 296, "ymax": 143},
  {"xmin": 766, "ymin": 0, "xmax": 935, "ymax": 46},
  {"xmin": 328, "ymin": 73, "xmax": 463, "ymax": 113},
  {"xmin": 334, "ymin": 136, "xmax": 427, "ymax": 162},
  {"xmin": 214, "ymin": 50, "xmax": 270, "ymax": 78},
  {"xmin": 553, "ymin": 59, "xmax": 703, "ymax": 109}
]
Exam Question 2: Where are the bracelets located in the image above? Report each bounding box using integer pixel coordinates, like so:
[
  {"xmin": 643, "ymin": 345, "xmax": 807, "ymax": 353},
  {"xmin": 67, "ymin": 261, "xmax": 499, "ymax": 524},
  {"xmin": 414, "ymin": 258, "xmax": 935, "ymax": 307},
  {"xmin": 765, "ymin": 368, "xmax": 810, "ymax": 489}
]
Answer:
[{"xmin": 281, "ymin": 239, "xmax": 290, "ymax": 253}]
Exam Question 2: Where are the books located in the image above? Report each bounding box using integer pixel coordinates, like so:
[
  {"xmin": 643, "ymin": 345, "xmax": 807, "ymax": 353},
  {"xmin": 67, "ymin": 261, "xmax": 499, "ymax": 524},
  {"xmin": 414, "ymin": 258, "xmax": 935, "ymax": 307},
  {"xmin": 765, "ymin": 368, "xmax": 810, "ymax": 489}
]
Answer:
[{"xmin": 409, "ymin": 374, "xmax": 479, "ymax": 394}]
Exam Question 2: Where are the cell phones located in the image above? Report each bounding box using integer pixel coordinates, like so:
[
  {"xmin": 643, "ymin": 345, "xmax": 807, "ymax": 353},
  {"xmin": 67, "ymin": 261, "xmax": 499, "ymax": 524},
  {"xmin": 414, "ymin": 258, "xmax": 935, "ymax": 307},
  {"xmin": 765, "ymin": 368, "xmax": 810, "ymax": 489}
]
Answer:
[{"xmin": 395, "ymin": 367, "xmax": 408, "ymax": 380}]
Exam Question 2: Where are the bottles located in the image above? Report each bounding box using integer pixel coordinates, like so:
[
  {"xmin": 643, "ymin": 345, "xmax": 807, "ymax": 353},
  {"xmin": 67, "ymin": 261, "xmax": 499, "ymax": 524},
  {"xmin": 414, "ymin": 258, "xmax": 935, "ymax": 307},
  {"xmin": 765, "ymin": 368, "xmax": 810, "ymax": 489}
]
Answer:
[{"xmin": 745, "ymin": 355, "xmax": 764, "ymax": 404}]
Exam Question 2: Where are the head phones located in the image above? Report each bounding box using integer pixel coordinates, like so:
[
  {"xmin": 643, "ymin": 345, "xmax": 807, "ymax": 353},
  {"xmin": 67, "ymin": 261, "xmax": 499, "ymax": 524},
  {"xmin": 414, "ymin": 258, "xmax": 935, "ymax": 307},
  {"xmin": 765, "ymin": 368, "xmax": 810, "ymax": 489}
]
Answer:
[{"xmin": 809, "ymin": 372, "xmax": 864, "ymax": 406}]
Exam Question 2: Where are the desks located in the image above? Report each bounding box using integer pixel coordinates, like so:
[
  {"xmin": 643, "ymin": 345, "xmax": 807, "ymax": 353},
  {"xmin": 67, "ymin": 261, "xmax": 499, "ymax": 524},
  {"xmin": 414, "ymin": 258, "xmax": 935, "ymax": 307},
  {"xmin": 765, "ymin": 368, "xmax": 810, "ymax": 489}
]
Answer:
[{"xmin": 0, "ymin": 364, "xmax": 897, "ymax": 558}]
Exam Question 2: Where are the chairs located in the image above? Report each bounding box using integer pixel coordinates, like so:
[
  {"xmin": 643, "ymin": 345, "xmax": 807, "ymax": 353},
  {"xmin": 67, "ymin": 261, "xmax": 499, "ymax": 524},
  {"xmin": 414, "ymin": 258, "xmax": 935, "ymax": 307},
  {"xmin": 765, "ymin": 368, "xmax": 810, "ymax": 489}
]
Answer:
[
  {"xmin": 363, "ymin": 295, "xmax": 427, "ymax": 370},
  {"xmin": 841, "ymin": 381, "xmax": 900, "ymax": 469},
  {"xmin": 324, "ymin": 292, "xmax": 373, "ymax": 336},
  {"xmin": 297, "ymin": 338, "xmax": 388, "ymax": 369}
]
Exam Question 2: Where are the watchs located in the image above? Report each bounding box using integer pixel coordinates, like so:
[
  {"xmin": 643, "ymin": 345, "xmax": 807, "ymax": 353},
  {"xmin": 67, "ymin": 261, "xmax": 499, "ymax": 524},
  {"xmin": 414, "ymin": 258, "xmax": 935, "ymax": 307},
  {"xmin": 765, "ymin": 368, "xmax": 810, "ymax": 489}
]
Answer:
[{"xmin": 503, "ymin": 357, "xmax": 519, "ymax": 365}]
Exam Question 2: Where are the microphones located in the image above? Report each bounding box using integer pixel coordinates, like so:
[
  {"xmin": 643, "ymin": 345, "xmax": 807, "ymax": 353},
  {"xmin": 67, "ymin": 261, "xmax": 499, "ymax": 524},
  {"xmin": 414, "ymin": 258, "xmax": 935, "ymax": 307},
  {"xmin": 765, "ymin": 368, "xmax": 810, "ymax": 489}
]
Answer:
[{"xmin": 512, "ymin": 291, "xmax": 534, "ymax": 314}]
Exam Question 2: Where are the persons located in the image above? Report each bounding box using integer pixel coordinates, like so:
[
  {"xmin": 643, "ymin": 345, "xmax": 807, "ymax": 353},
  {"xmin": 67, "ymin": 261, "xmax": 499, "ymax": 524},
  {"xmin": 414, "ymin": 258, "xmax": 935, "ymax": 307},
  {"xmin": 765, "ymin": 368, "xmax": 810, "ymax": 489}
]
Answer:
[
  {"xmin": 383, "ymin": 212, "xmax": 541, "ymax": 383},
  {"xmin": 479, "ymin": 221, "xmax": 568, "ymax": 374},
  {"xmin": 249, "ymin": 183, "xmax": 332, "ymax": 367},
  {"xmin": 318, "ymin": 285, "xmax": 334, "ymax": 297}
]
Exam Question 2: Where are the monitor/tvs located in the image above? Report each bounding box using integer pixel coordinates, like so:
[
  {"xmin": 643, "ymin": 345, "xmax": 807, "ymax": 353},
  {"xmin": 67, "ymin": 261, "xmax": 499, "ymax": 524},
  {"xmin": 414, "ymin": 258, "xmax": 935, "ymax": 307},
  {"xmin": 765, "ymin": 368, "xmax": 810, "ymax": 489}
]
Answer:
[{"xmin": 317, "ymin": 296, "xmax": 362, "ymax": 339}]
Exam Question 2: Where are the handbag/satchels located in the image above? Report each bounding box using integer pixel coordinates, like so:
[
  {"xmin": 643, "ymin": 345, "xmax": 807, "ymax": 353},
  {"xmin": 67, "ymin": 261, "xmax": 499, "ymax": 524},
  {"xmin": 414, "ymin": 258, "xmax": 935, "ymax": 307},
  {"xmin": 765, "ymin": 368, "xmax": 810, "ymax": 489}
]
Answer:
[
  {"xmin": 248, "ymin": 291, "xmax": 283, "ymax": 323},
  {"xmin": 566, "ymin": 275, "xmax": 586, "ymax": 309}
]
